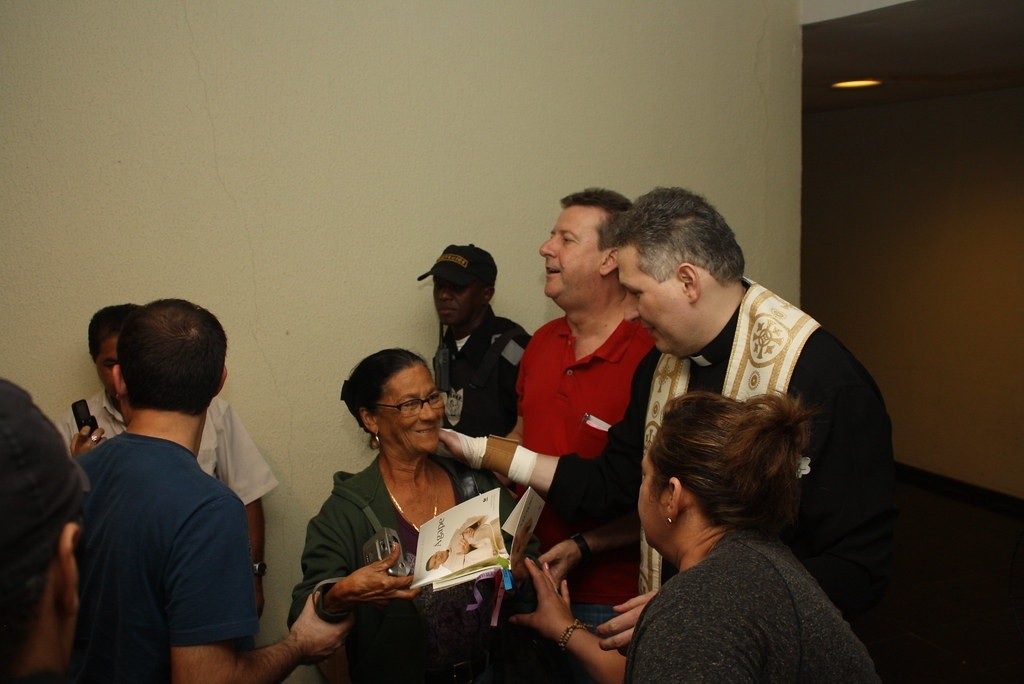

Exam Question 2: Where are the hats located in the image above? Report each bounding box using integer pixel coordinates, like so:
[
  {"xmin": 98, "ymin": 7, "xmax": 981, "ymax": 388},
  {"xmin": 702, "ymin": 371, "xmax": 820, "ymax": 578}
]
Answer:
[
  {"xmin": 416, "ymin": 244, "xmax": 497, "ymax": 287},
  {"xmin": 0, "ymin": 379, "xmax": 92, "ymax": 595}
]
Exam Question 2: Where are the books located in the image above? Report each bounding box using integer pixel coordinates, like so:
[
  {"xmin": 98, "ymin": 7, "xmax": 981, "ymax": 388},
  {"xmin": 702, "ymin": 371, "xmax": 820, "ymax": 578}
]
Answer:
[{"xmin": 410, "ymin": 485, "xmax": 546, "ymax": 592}]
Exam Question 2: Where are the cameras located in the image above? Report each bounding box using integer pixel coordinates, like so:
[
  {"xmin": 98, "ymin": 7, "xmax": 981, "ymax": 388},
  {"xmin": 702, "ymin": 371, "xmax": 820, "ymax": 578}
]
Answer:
[{"xmin": 361, "ymin": 528, "xmax": 409, "ymax": 577}]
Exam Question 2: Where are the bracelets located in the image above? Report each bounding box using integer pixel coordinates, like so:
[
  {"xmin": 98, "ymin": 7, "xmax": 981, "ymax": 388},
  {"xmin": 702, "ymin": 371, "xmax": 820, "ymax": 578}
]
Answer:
[
  {"xmin": 558, "ymin": 619, "xmax": 593, "ymax": 654},
  {"xmin": 571, "ymin": 533, "xmax": 591, "ymax": 557}
]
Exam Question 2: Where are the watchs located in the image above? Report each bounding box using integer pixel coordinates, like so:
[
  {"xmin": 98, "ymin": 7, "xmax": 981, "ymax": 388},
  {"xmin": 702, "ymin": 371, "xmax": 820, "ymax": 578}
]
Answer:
[{"xmin": 254, "ymin": 562, "xmax": 267, "ymax": 576}]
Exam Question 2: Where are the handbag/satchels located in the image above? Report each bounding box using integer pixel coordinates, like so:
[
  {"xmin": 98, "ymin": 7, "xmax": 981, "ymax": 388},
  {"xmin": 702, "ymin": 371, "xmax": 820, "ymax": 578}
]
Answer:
[{"xmin": 495, "ymin": 638, "xmax": 573, "ymax": 684}]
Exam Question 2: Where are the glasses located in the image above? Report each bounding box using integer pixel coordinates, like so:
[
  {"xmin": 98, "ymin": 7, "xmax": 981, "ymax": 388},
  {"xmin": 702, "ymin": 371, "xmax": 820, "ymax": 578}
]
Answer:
[{"xmin": 372, "ymin": 391, "xmax": 448, "ymax": 417}]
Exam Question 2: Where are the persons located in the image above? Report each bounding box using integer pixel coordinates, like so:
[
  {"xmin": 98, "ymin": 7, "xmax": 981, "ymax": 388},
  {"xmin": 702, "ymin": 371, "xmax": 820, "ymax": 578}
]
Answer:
[
  {"xmin": 0, "ymin": 378, "xmax": 96, "ymax": 684},
  {"xmin": 72, "ymin": 300, "xmax": 354, "ymax": 684},
  {"xmin": 57, "ymin": 304, "xmax": 279, "ymax": 622},
  {"xmin": 286, "ymin": 349, "xmax": 542, "ymax": 684},
  {"xmin": 418, "ymin": 244, "xmax": 534, "ymax": 437},
  {"xmin": 509, "ymin": 391, "xmax": 884, "ymax": 684},
  {"xmin": 436, "ymin": 186, "xmax": 894, "ymax": 652},
  {"xmin": 495, "ymin": 188, "xmax": 657, "ymax": 684}
]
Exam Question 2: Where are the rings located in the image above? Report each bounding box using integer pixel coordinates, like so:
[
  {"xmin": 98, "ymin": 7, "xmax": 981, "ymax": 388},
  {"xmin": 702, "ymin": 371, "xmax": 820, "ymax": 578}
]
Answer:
[{"xmin": 91, "ymin": 436, "xmax": 97, "ymax": 441}]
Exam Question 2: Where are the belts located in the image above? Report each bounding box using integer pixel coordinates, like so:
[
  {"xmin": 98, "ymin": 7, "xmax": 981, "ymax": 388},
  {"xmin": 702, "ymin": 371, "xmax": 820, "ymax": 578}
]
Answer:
[{"xmin": 427, "ymin": 646, "xmax": 501, "ymax": 684}]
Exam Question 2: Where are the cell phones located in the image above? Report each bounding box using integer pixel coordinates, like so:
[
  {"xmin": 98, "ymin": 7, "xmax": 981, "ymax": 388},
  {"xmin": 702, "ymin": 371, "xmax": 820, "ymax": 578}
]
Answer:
[{"xmin": 71, "ymin": 398, "xmax": 98, "ymax": 439}]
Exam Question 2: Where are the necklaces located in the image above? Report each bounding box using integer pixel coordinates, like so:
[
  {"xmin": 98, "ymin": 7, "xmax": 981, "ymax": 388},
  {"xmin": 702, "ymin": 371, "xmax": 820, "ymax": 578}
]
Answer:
[{"xmin": 386, "ymin": 478, "xmax": 440, "ymax": 533}]
